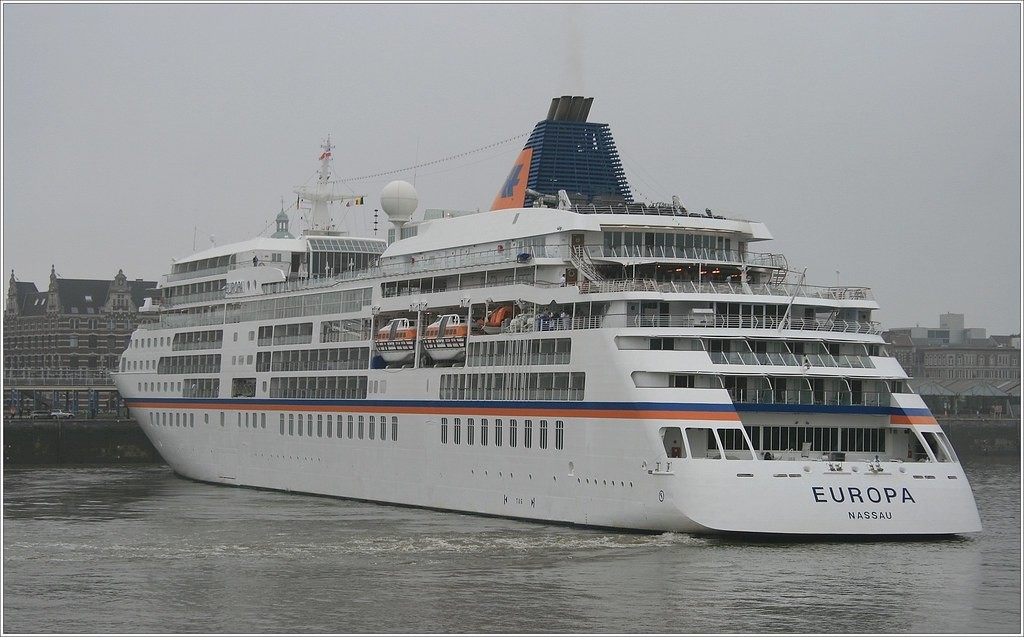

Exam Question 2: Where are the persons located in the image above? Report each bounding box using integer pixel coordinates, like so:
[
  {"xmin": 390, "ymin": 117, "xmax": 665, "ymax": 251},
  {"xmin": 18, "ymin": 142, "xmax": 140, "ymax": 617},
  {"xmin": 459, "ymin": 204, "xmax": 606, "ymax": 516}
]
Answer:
[
  {"xmin": 560, "ymin": 274, "xmax": 565, "ymax": 288},
  {"xmin": 873, "ymin": 455, "xmax": 880, "ymax": 462},
  {"xmin": 253, "ymin": 255, "xmax": 258, "ymax": 267},
  {"xmin": 537, "ymin": 309, "xmax": 586, "ymax": 331}
]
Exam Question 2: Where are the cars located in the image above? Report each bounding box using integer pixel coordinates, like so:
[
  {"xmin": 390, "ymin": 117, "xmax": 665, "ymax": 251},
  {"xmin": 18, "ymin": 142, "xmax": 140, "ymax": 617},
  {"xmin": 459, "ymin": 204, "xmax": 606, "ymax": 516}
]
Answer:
[
  {"xmin": 51, "ymin": 409, "xmax": 76, "ymax": 420},
  {"xmin": 30, "ymin": 410, "xmax": 52, "ymax": 419}
]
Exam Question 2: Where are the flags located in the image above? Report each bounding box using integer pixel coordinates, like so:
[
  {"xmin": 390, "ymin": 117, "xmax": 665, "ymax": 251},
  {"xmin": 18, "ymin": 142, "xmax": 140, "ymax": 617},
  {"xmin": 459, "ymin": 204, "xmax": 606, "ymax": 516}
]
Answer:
[
  {"xmin": 317, "ymin": 145, "xmax": 331, "ymax": 161},
  {"xmin": 346, "ymin": 197, "xmax": 364, "ymax": 207}
]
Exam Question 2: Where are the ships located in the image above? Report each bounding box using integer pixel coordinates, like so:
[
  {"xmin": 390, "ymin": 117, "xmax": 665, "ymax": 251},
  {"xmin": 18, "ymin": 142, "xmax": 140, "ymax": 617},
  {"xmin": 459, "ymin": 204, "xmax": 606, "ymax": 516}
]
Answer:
[{"xmin": 108, "ymin": 95, "xmax": 983, "ymax": 536}]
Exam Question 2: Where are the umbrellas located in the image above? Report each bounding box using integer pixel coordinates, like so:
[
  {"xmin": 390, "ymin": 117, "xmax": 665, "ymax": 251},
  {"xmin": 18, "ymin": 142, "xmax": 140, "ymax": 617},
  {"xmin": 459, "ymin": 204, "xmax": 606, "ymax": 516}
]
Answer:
[{"xmin": 908, "ymin": 381, "xmax": 1011, "ymax": 417}]
